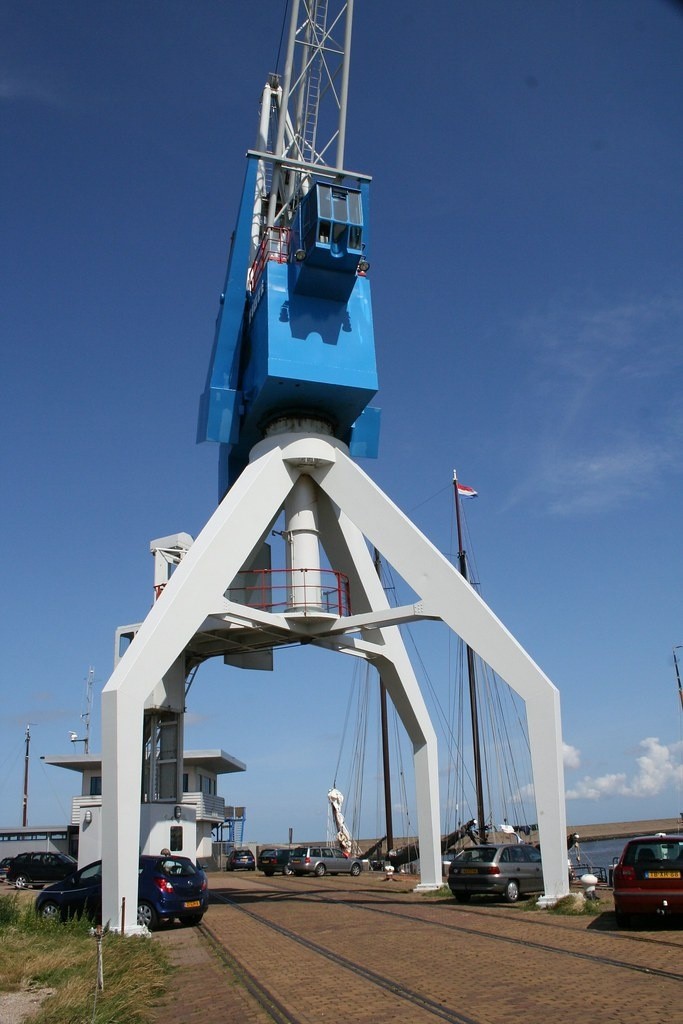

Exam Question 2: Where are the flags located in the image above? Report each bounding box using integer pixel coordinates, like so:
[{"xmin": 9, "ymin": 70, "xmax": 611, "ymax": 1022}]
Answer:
[{"xmin": 457, "ymin": 483, "xmax": 478, "ymax": 500}]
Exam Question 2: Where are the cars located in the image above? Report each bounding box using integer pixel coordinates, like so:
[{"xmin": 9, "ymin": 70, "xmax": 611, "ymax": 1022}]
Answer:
[
  {"xmin": 256, "ymin": 848, "xmax": 294, "ymax": 877},
  {"xmin": 225, "ymin": 850, "xmax": 255, "ymax": 871},
  {"xmin": 0, "ymin": 857, "xmax": 14, "ymax": 882},
  {"xmin": 287, "ymin": 845, "xmax": 363, "ymax": 877},
  {"xmin": 5, "ymin": 852, "xmax": 78, "ymax": 890},
  {"xmin": 445, "ymin": 843, "xmax": 545, "ymax": 904},
  {"xmin": 34, "ymin": 855, "xmax": 209, "ymax": 930}
]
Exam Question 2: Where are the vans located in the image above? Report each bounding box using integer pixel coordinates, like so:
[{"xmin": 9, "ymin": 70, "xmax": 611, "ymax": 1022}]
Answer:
[{"xmin": 612, "ymin": 834, "xmax": 683, "ymax": 928}]
